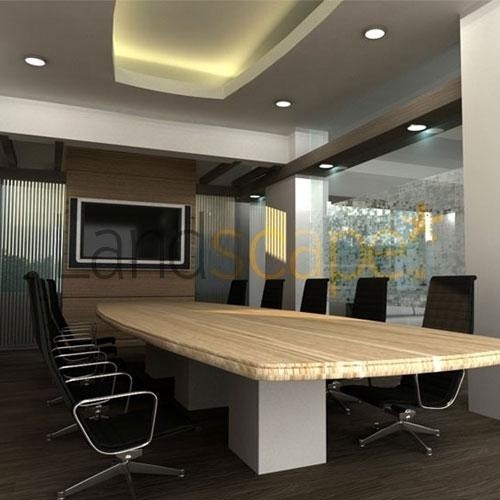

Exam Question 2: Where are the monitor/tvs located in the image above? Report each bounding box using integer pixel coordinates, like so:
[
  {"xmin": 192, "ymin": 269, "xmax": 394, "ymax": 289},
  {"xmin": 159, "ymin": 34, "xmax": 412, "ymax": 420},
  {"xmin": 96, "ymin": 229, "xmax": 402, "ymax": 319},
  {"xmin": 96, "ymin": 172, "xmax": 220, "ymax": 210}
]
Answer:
[{"xmin": 76, "ymin": 197, "xmax": 185, "ymax": 265}]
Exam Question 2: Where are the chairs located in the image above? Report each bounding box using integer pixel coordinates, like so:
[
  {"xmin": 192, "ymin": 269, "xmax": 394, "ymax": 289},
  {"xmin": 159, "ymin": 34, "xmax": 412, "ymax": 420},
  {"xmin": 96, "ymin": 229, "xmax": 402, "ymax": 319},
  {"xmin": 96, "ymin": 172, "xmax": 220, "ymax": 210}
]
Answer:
[
  {"xmin": 226, "ymin": 280, "xmax": 248, "ymax": 305},
  {"xmin": 260, "ymin": 279, "xmax": 286, "ymax": 310},
  {"xmin": 299, "ymin": 278, "xmax": 329, "ymax": 315},
  {"xmin": 23, "ymin": 269, "xmax": 185, "ymax": 500},
  {"xmin": 350, "ymin": 275, "xmax": 389, "ymax": 325},
  {"xmin": 332, "ymin": 275, "xmax": 477, "ymax": 457}
]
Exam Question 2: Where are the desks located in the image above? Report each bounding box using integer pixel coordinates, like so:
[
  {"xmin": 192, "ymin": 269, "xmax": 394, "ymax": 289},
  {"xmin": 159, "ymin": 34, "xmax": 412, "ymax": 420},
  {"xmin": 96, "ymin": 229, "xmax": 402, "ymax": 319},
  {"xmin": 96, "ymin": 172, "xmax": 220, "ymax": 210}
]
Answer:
[{"xmin": 95, "ymin": 299, "xmax": 500, "ymax": 475}]
